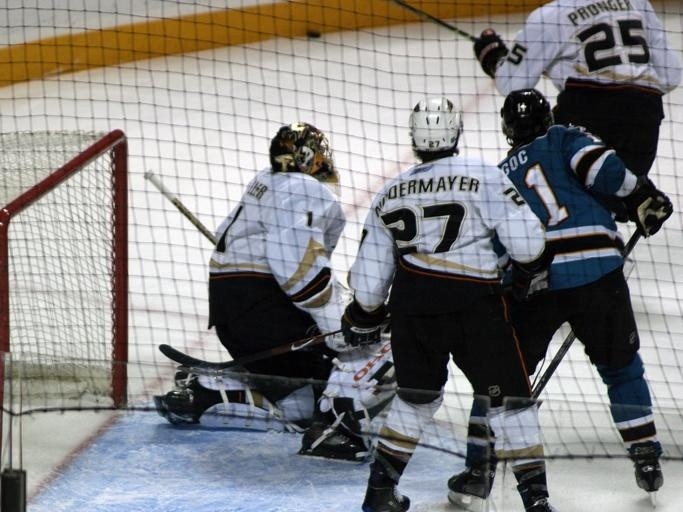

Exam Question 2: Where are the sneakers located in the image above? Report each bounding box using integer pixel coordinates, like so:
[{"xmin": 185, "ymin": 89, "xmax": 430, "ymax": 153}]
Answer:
[
  {"xmin": 153, "ymin": 388, "xmax": 203, "ymax": 428},
  {"xmin": 302, "ymin": 416, "xmax": 374, "ymax": 461}
]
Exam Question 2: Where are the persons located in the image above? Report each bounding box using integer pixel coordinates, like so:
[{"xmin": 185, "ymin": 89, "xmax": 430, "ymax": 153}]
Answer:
[
  {"xmin": 446, "ymin": 87, "xmax": 677, "ymax": 499},
  {"xmin": 153, "ymin": 119, "xmax": 376, "ymax": 464},
  {"xmin": 339, "ymin": 95, "xmax": 554, "ymax": 511},
  {"xmin": 472, "ymin": 1, "xmax": 682, "ymax": 224}
]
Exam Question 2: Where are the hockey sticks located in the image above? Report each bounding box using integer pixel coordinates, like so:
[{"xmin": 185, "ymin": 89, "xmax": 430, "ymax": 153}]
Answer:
[{"xmin": 159, "ymin": 336, "xmax": 326, "ymax": 369}]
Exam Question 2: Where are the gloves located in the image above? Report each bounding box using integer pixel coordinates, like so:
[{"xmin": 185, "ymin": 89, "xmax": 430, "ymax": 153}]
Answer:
[
  {"xmin": 340, "ymin": 296, "xmax": 388, "ymax": 347},
  {"xmin": 473, "ymin": 26, "xmax": 509, "ymax": 80},
  {"xmin": 624, "ymin": 178, "xmax": 674, "ymax": 240},
  {"xmin": 512, "ymin": 242, "xmax": 555, "ymax": 302}
]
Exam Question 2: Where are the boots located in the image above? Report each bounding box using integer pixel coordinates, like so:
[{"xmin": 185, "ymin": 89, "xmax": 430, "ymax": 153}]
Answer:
[
  {"xmin": 362, "ymin": 462, "xmax": 411, "ymax": 512},
  {"xmin": 516, "ymin": 481, "xmax": 553, "ymax": 512},
  {"xmin": 628, "ymin": 440, "xmax": 664, "ymax": 491},
  {"xmin": 447, "ymin": 456, "xmax": 501, "ymax": 500}
]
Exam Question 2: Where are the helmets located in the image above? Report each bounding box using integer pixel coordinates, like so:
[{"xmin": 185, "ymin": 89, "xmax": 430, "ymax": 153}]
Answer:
[
  {"xmin": 269, "ymin": 122, "xmax": 341, "ymax": 184},
  {"xmin": 499, "ymin": 86, "xmax": 553, "ymax": 146},
  {"xmin": 406, "ymin": 94, "xmax": 464, "ymax": 161}
]
